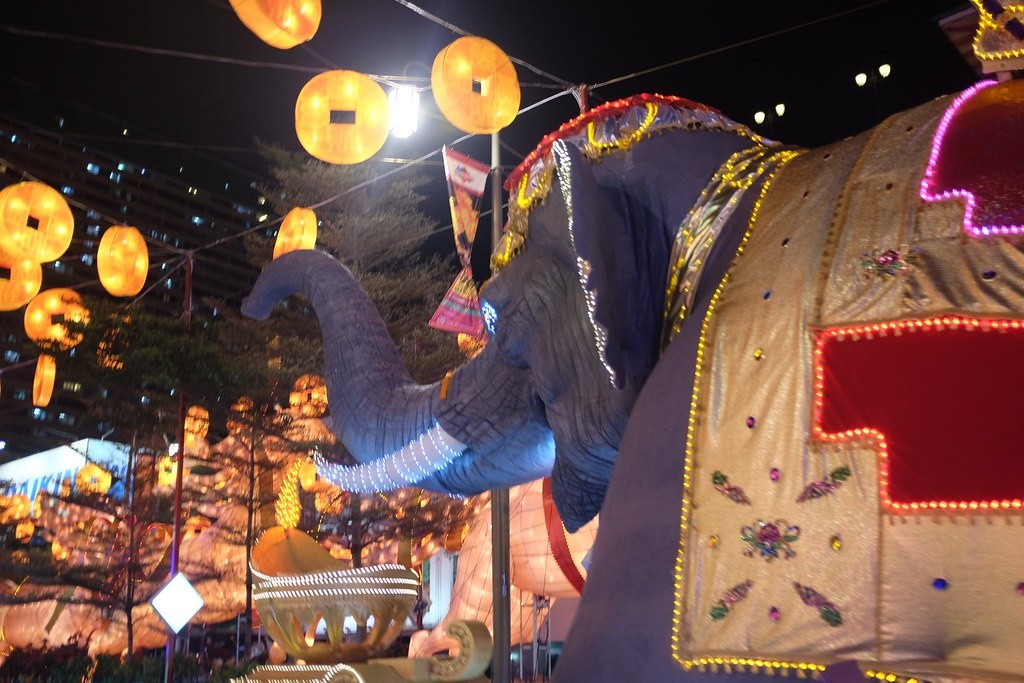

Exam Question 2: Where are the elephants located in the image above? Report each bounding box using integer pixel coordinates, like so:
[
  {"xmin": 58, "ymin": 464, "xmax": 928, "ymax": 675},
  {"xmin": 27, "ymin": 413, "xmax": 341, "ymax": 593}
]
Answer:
[{"xmin": 241, "ymin": 78, "xmax": 1024, "ymax": 683}]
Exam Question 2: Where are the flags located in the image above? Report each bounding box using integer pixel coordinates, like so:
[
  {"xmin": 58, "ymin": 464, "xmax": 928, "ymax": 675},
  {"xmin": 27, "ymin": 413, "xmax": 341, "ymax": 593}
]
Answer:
[{"xmin": 427, "ymin": 144, "xmax": 492, "ymax": 338}]
